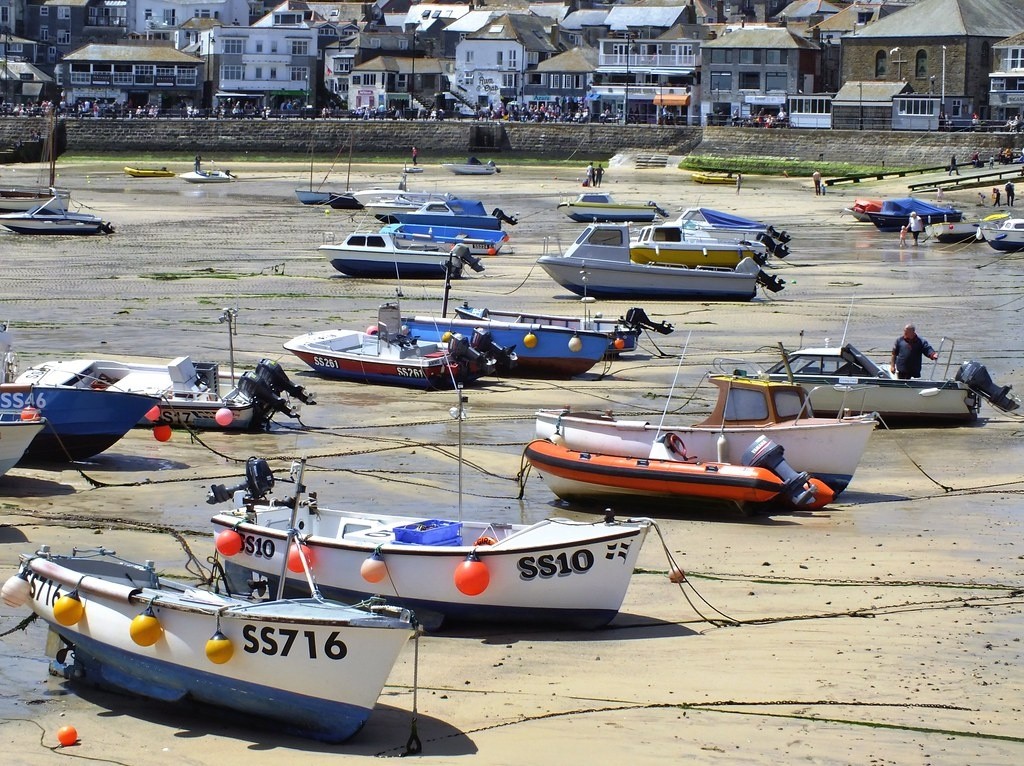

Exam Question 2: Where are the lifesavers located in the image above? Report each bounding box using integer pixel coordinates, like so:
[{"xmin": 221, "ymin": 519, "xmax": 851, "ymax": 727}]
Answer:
[{"xmin": 471, "ymin": 536, "xmax": 498, "ymax": 546}]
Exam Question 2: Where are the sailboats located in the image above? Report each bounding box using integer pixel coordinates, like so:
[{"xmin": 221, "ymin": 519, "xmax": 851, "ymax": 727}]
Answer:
[{"xmin": 0, "ymin": 101, "xmax": 71, "ymax": 212}]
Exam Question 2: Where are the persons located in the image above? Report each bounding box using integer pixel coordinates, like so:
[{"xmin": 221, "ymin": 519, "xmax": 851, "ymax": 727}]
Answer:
[
  {"xmin": 937, "ymin": 186, "xmax": 943, "ymax": 201},
  {"xmin": 938, "ymin": 111, "xmax": 953, "ymax": 132},
  {"xmin": 586, "ymin": 162, "xmax": 595, "ymax": 186},
  {"xmin": 727, "ymin": 173, "xmax": 732, "ymax": 178},
  {"xmin": 736, "ymin": 173, "xmax": 742, "ymax": 194},
  {"xmin": 949, "ymin": 148, "xmax": 1024, "ymax": 207},
  {"xmin": 891, "ymin": 324, "xmax": 938, "ymax": 379},
  {"xmin": 595, "ymin": 164, "xmax": 605, "ymax": 188},
  {"xmin": 0, "ymin": 101, "xmax": 789, "ymax": 128},
  {"xmin": 1006, "ymin": 113, "xmax": 1020, "ymax": 132},
  {"xmin": 822, "ymin": 185, "xmax": 825, "ymax": 195},
  {"xmin": 232, "ymin": 18, "xmax": 240, "ymax": 26},
  {"xmin": 972, "ymin": 111, "xmax": 980, "ymax": 119},
  {"xmin": 813, "ymin": 169, "xmax": 820, "ymax": 195},
  {"xmin": 195, "ymin": 152, "xmax": 201, "ymax": 173},
  {"xmin": 899, "ymin": 212, "xmax": 923, "ymax": 248},
  {"xmin": 412, "ymin": 147, "xmax": 417, "ymax": 165}
]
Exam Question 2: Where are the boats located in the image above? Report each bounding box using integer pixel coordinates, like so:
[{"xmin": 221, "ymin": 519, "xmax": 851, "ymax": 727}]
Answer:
[
  {"xmin": 844, "ymin": 197, "xmax": 883, "ymax": 223},
  {"xmin": 711, "ymin": 291, "xmax": 1024, "ymax": 429},
  {"xmin": 978, "ymin": 211, "xmax": 1024, "ymax": 253},
  {"xmin": 533, "ymin": 340, "xmax": 881, "ymax": 500},
  {"xmin": 291, "ymin": 124, "xmax": 793, "ymax": 382},
  {"xmin": 864, "ymin": 196, "xmax": 967, "ymax": 232},
  {"xmin": 282, "ymin": 285, "xmax": 518, "ymax": 393},
  {"xmin": 524, "ymin": 430, "xmax": 838, "ymax": 522},
  {"xmin": 1, "ymin": 451, "xmax": 419, "ymax": 747},
  {"xmin": 0, "ymin": 304, "xmax": 319, "ymax": 480},
  {"xmin": 924, "ymin": 213, "xmax": 1002, "ymax": 244},
  {"xmin": 124, "ymin": 165, "xmax": 175, "ymax": 177},
  {"xmin": 0, "ymin": 183, "xmax": 116, "ymax": 236},
  {"xmin": 203, "ymin": 384, "xmax": 655, "ymax": 635},
  {"xmin": 178, "ymin": 166, "xmax": 240, "ymax": 183}
]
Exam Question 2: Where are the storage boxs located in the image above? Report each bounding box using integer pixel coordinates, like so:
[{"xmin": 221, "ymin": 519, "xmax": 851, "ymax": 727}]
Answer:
[
  {"xmin": 392, "ymin": 519, "xmax": 464, "ymax": 544},
  {"xmin": 391, "ymin": 536, "xmax": 463, "ymax": 546}
]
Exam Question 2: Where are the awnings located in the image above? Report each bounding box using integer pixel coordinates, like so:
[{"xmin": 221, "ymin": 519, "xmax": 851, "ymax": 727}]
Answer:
[
  {"xmin": 744, "ymin": 96, "xmax": 786, "ymax": 105},
  {"xmin": 585, "ymin": 94, "xmax": 601, "ymax": 101},
  {"xmin": 215, "ymin": 93, "xmax": 264, "ymax": 101},
  {"xmin": 653, "ymin": 94, "xmax": 690, "ymax": 106}
]
